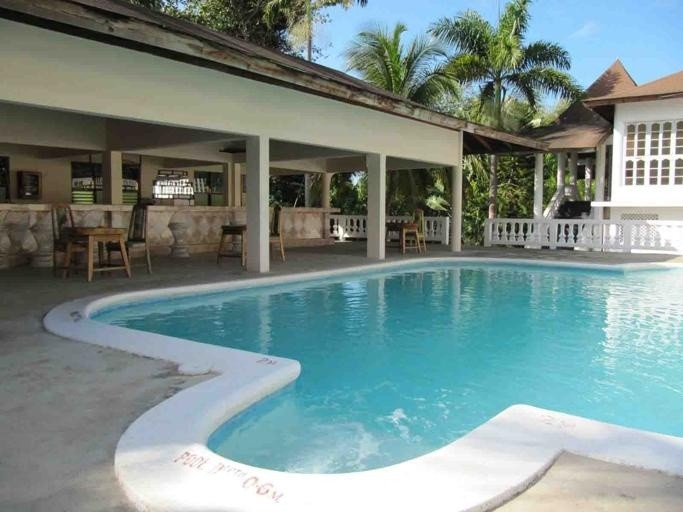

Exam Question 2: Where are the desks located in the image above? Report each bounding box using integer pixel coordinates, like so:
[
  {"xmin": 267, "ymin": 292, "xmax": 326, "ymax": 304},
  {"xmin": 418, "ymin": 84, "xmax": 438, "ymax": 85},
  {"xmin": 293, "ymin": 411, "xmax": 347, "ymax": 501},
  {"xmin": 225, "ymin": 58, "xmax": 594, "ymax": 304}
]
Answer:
[
  {"xmin": 385, "ymin": 222, "xmax": 422, "ymax": 254},
  {"xmin": 216, "ymin": 223, "xmax": 246, "ymax": 265},
  {"xmin": 60, "ymin": 227, "xmax": 132, "ymax": 282}
]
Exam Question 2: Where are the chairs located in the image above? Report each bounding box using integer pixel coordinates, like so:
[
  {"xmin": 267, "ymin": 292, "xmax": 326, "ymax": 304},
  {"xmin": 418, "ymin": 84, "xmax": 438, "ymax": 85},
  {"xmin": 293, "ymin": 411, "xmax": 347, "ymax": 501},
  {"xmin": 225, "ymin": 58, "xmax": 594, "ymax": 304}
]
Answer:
[
  {"xmin": 400, "ymin": 209, "xmax": 427, "ymax": 252},
  {"xmin": 269, "ymin": 203, "xmax": 286, "ymax": 262},
  {"xmin": 49, "ymin": 202, "xmax": 152, "ymax": 277}
]
